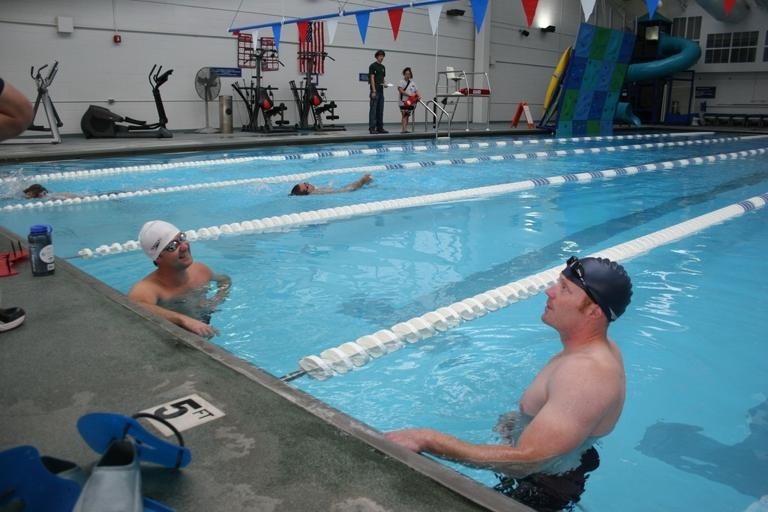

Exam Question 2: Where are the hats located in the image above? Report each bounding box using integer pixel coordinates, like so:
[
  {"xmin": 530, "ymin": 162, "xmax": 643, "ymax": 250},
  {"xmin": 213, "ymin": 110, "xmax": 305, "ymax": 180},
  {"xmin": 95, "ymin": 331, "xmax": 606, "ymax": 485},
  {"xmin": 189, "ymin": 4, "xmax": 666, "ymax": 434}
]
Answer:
[
  {"xmin": 374, "ymin": 49, "xmax": 385, "ymax": 59},
  {"xmin": 137, "ymin": 219, "xmax": 180, "ymax": 259},
  {"xmin": 561, "ymin": 255, "xmax": 633, "ymax": 325}
]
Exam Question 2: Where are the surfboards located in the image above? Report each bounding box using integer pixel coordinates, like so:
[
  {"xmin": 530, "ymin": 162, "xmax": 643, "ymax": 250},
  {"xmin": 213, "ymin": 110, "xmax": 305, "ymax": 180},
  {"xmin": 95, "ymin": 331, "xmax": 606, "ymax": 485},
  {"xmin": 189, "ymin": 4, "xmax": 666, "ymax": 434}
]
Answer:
[{"xmin": 543, "ymin": 46, "xmax": 571, "ymax": 113}]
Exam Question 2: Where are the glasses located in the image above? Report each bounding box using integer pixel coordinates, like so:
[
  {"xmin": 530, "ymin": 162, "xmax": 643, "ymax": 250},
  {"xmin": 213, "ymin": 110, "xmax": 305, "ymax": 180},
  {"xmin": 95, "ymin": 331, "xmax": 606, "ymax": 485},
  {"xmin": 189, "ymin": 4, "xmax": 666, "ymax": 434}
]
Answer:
[
  {"xmin": 163, "ymin": 233, "xmax": 186, "ymax": 252},
  {"xmin": 567, "ymin": 254, "xmax": 594, "ymax": 305},
  {"xmin": 302, "ymin": 182, "xmax": 309, "ymax": 193}
]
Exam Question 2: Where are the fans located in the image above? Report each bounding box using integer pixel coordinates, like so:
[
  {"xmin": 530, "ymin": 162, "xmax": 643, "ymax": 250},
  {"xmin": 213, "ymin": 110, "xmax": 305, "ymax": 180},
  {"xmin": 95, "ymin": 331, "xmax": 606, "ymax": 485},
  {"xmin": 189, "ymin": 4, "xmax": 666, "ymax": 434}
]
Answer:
[{"xmin": 194, "ymin": 67, "xmax": 220, "ymax": 134}]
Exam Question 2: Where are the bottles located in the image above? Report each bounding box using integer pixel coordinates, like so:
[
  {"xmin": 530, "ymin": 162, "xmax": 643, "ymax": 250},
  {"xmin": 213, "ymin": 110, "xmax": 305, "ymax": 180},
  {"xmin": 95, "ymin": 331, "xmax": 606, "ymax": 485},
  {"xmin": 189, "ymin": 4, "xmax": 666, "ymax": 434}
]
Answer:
[{"xmin": 28, "ymin": 224, "xmax": 55, "ymax": 276}]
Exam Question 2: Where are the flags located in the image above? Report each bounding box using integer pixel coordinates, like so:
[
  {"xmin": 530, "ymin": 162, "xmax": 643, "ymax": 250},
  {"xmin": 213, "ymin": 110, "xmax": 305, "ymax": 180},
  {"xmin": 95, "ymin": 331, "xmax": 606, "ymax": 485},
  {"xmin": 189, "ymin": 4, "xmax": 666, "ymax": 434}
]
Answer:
[{"xmin": 299, "ymin": 20, "xmax": 324, "ymax": 74}]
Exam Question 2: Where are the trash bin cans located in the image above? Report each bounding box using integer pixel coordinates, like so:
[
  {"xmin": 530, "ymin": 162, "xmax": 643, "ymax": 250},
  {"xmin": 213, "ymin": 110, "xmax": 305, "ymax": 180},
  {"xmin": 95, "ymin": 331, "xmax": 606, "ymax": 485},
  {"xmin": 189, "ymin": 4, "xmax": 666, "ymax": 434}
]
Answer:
[{"xmin": 219, "ymin": 95, "xmax": 233, "ymax": 134}]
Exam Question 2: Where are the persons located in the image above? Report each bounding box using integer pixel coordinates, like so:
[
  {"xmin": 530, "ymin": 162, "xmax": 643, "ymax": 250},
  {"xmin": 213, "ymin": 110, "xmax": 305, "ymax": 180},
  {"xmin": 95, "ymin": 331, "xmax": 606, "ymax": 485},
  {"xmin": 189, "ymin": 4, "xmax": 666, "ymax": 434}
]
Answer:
[
  {"xmin": 127, "ymin": 219, "xmax": 232, "ymax": 341},
  {"xmin": 368, "ymin": 50, "xmax": 389, "ymax": 134},
  {"xmin": 290, "ymin": 173, "xmax": 372, "ymax": 194},
  {"xmin": 0, "ymin": 77, "xmax": 34, "ymax": 334},
  {"xmin": 22, "ymin": 184, "xmax": 48, "ymax": 199},
  {"xmin": 397, "ymin": 67, "xmax": 420, "ymax": 133},
  {"xmin": 383, "ymin": 256, "xmax": 633, "ymax": 511}
]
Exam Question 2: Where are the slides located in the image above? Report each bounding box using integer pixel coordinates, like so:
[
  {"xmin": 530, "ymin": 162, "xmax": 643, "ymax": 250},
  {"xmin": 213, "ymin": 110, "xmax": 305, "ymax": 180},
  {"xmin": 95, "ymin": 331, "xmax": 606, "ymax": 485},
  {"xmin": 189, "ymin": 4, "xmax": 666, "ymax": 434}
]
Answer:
[{"xmin": 614, "ymin": 32, "xmax": 701, "ymax": 128}]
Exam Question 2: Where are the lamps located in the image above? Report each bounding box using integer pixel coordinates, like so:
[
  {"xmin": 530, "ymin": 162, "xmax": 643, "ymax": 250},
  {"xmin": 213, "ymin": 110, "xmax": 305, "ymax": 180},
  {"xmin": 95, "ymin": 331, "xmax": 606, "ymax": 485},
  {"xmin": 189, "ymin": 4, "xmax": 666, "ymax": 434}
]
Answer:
[
  {"xmin": 447, "ymin": 9, "xmax": 465, "ymax": 16},
  {"xmin": 541, "ymin": 26, "xmax": 555, "ymax": 33}
]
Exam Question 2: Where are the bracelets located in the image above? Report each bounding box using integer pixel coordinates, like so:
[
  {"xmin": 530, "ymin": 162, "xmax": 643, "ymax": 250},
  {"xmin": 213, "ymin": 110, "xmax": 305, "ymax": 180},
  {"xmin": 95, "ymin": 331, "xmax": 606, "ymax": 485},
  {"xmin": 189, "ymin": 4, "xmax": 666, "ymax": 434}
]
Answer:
[{"xmin": 372, "ymin": 92, "xmax": 376, "ymax": 93}]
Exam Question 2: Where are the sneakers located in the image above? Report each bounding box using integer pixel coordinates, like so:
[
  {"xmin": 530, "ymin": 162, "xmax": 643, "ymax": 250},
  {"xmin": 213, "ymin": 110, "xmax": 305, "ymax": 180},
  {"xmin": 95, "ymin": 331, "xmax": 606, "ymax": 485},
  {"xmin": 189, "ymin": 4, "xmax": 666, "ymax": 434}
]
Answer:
[
  {"xmin": 370, "ymin": 127, "xmax": 412, "ymax": 135},
  {"xmin": 1, "ymin": 306, "xmax": 27, "ymax": 332}
]
